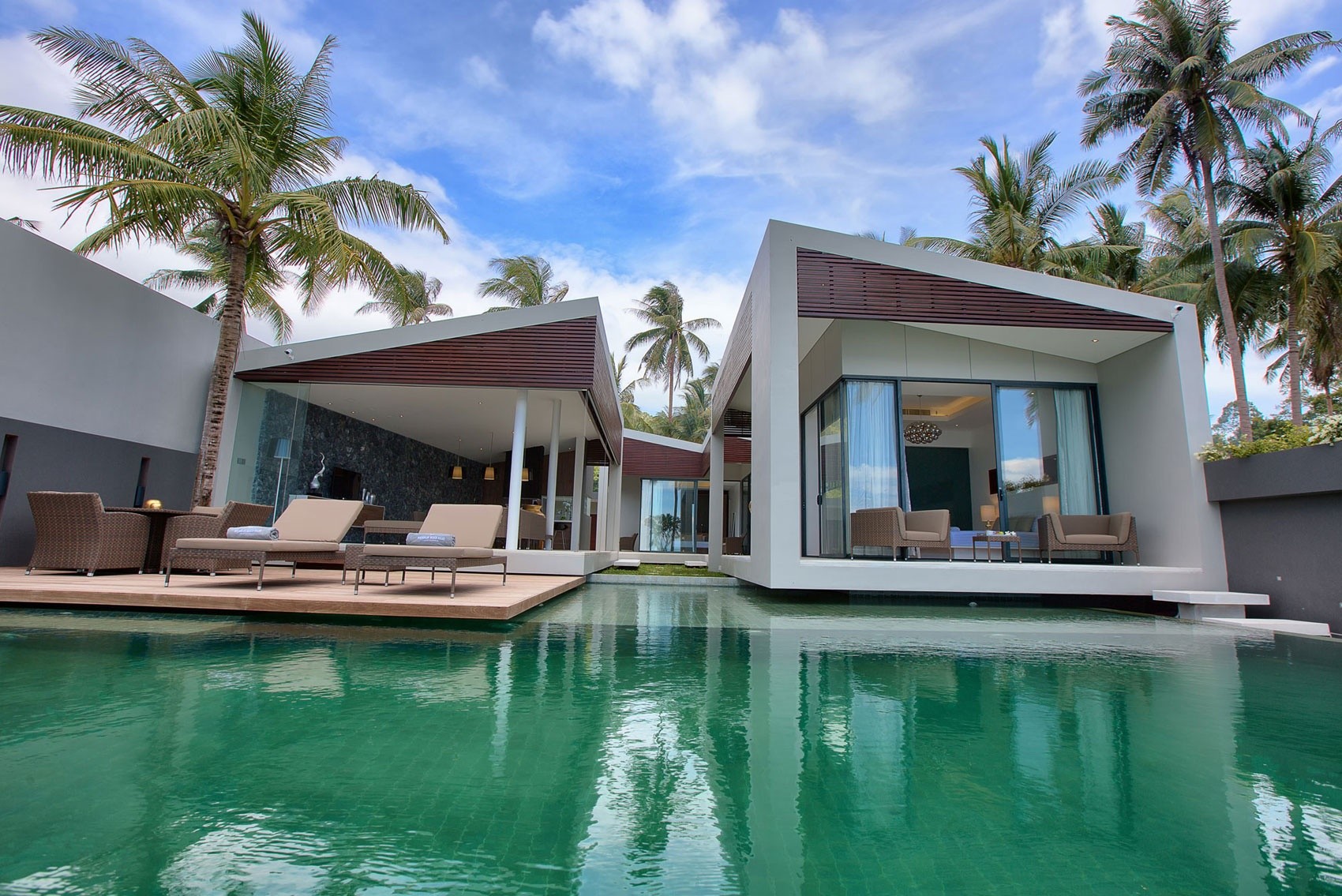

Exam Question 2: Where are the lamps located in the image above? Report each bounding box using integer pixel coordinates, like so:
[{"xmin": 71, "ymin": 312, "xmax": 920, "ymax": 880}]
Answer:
[
  {"xmin": 484, "ymin": 431, "xmax": 494, "ymax": 480},
  {"xmin": 273, "ymin": 439, "xmax": 292, "ymax": 525},
  {"xmin": 452, "ymin": 436, "xmax": 463, "ymax": 479},
  {"xmin": 980, "ymin": 505, "xmax": 998, "ymax": 535},
  {"xmin": 902, "ymin": 394, "xmax": 942, "ymax": 444},
  {"xmin": 521, "ymin": 426, "xmax": 528, "ymax": 481}
]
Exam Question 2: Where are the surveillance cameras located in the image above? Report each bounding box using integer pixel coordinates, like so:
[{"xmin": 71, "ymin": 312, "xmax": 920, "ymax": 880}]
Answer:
[
  {"xmin": 284, "ymin": 348, "xmax": 292, "ymax": 356},
  {"xmin": 1173, "ymin": 305, "xmax": 1183, "ymax": 311}
]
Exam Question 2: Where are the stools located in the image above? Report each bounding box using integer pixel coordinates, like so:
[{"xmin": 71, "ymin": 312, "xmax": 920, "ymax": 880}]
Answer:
[{"xmin": 553, "ymin": 523, "xmax": 568, "ymax": 550}]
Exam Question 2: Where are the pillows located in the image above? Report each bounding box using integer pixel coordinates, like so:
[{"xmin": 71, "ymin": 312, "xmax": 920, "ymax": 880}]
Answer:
[{"xmin": 992, "ymin": 515, "xmax": 1040, "ymax": 532}]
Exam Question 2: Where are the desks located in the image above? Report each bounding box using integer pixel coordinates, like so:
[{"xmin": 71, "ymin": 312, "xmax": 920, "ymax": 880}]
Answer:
[
  {"xmin": 972, "ymin": 535, "xmax": 1022, "ymax": 564},
  {"xmin": 103, "ymin": 506, "xmax": 219, "ymax": 574}
]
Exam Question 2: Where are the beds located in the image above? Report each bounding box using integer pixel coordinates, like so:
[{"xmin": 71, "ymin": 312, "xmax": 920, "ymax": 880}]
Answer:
[{"xmin": 908, "ymin": 526, "xmax": 1041, "ymax": 561}]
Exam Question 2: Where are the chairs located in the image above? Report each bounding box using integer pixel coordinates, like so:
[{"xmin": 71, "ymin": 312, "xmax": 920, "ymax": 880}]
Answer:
[
  {"xmin": 352, "ymin": 504, "xmax": 507, "ymax": 596},
  {"xmin": 24, "ymin": 489, "xmax": 153, "ymax": 577},
  {"xmin": 851, "ymin": 506, "xmax": 951, "ymax": 562},
  {"xmin": 164, "ymin": 499, "xmax": 365, "ymax": 590},
  {"xmin": 1038, "ymin": 512, "xmax": 1140, "ymax": 567},
  {"xmin": 160, "ymin": 502, "xmax": 274, "ymax": 576},
  {"xmin": 725, "ymin": 532, "xmax": 748, "ymax": 555},
  {"xmin": 620, "ymin": 533, "xmax": 638, "ymax": 551}
]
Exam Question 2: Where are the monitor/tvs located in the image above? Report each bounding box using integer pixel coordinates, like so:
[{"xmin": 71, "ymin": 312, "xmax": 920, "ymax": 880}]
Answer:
[{"xmin": 329, "ymin": 466, "xmax": 362, "ymax": 501}]
